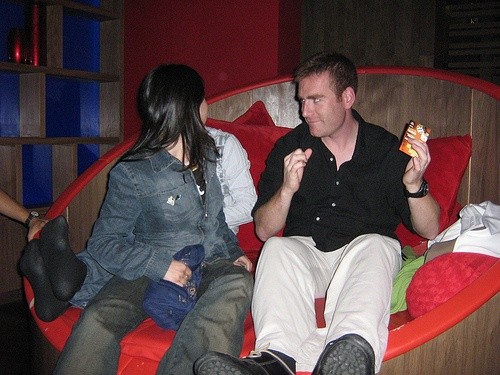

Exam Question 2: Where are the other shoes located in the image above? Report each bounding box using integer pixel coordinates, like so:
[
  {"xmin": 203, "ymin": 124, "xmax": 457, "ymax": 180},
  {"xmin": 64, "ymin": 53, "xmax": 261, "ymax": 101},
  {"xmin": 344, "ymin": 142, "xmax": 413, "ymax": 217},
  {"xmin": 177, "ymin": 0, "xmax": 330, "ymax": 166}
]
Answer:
[
  {"xmin": 192, "ymin": 342, "xmax": 297, "ymax": 375},
  {"xmin": 309, "ymin": 332, "xmax": 377, "ymax": 375}
]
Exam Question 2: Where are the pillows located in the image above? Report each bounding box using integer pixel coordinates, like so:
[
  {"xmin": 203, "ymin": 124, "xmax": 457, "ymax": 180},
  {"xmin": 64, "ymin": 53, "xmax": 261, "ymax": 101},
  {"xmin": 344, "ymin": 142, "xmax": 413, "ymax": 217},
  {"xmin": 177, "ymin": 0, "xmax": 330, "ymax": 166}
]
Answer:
[
  {"xmin": 207, "ymin": 102, "xmax": 292, "ymax": 252},
  {"xmin": 390, "ymin": 134, "xmax": 499, "ymax": 318}
]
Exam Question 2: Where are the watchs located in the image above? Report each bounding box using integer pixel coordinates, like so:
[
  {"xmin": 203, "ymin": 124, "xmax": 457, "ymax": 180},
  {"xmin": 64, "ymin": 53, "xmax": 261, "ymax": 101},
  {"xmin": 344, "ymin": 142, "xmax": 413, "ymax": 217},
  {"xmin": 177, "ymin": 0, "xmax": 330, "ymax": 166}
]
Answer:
[
  {"xmin": 407, "ymin": 181, "xmax": 429, "ymax": 198},
  {"xmin": 23, "ymin": 209, "xmax": 40, "ymax": 228}
]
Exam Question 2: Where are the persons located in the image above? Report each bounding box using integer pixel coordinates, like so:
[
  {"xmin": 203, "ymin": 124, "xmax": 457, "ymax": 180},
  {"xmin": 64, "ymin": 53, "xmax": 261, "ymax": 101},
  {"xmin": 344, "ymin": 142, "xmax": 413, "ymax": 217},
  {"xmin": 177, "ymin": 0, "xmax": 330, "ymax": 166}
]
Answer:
[
  {"xmin": 15, "ymin": 126, "xmax": 260, "ymax": 321},
  {"xmin": 0, "ymin": 188, "xmax": 47, "ymax": 242},
  {"xmin": 52, "ymin": 64, "xmax": 254, "ymax": 375},
  {"xmin": 191, "ymin": 51, "xmax": 441, "ymax": 375}
]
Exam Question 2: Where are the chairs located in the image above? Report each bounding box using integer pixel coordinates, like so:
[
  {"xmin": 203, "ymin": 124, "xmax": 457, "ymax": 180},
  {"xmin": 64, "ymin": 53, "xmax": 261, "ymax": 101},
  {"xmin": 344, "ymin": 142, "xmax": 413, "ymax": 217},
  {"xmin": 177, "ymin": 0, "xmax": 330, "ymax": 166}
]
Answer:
[{"xmin": 22, "ymin": 64, "xmax": 500, "ymax": 374}]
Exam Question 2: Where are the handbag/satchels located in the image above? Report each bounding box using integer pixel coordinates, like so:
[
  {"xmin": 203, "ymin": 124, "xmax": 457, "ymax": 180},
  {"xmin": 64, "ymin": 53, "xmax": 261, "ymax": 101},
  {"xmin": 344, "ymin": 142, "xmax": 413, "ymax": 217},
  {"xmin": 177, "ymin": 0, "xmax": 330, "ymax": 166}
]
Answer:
[{"xmin": 143, "ymin": 243, "xmax": 205, "ymax": 331}]
